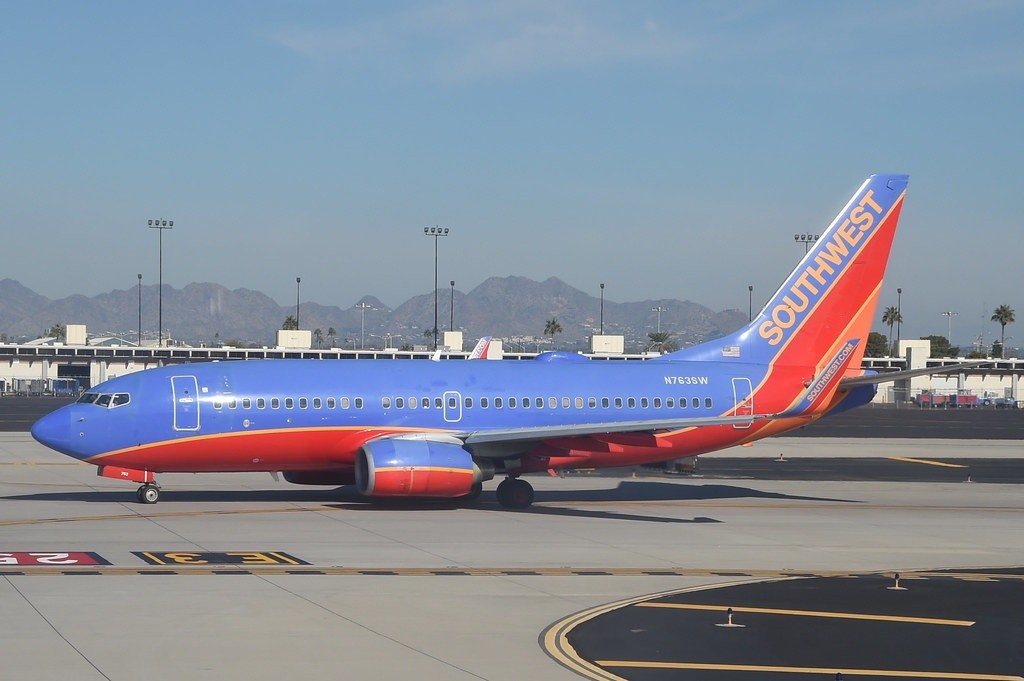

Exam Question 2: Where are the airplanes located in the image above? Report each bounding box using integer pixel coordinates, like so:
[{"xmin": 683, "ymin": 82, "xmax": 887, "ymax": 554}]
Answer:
[{"xmin": 28, "ymin": 174, "xmax": 909, "ymax": 517}]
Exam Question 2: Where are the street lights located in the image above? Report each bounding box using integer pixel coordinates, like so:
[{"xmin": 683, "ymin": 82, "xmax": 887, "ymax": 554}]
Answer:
[
  {"xmin": 296, "ymin": 277, "xmax": 302, "ymax": 331},
  {"xmin": 897, "ymin": 288, "xmax": 901, "ymax": 342},
  {"xmin": 600, "ymin": 284, "xmax": 604, "ymax": 335},
  {"xmin": 451, "ymin": 281, "xmax": 455, "ymax": 331},
  {"xmin": 423, "ymin": 225, "xmax": 449, "ymax": 352},
  {"xmin": 749, "ymin": 285, "xmax": 753, "ymax": 324},
  {"xmin": 941, "ymin": 312, "xmax": 960, "ymax": 344},
  {"xmin": 147, "ymin": 219, "xmax": 176, "ymax": 346},
  {"xmin": 138, "ymin": 273, "xmax": 142, "ymax": 347},
  {"xmin": 794, "ymin": 231, "xmax": 819, "ymax": 255}
]
